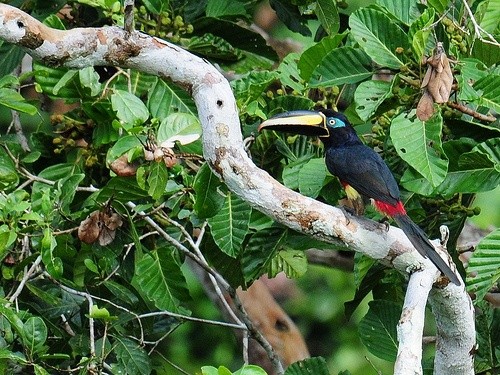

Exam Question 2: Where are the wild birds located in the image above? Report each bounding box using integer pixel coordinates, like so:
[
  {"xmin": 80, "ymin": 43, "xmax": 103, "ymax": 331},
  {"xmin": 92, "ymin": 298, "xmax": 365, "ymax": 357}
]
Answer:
[{"xmin": 258, "ymin": 109, "xmax": 461, "ymax": 287}]
